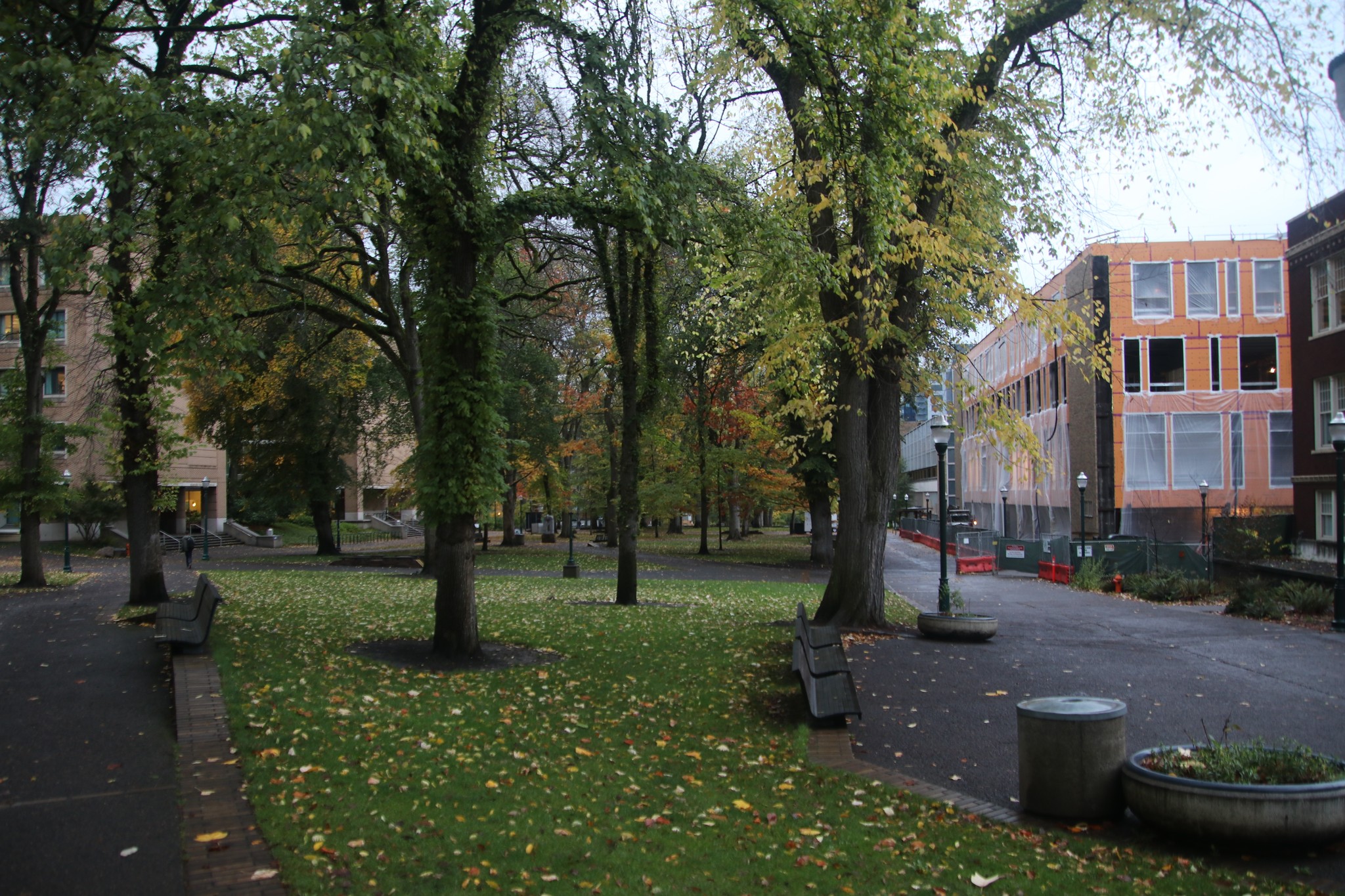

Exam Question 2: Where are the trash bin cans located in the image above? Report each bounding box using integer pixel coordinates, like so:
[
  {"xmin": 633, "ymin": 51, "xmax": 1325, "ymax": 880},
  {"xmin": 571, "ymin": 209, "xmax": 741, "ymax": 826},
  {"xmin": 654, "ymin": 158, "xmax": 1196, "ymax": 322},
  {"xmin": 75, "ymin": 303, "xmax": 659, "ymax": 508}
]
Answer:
[
  {"xmin": 514, "ymin": 534, "xmax": 525, "ymax": 545},
  {"xmin": 1017, "ymin": 697, "xmax": 1127, "ymax": 816},
  {"xmin": 1093, "ymin": 533, "xmax": 1138, "ymax": 540}
]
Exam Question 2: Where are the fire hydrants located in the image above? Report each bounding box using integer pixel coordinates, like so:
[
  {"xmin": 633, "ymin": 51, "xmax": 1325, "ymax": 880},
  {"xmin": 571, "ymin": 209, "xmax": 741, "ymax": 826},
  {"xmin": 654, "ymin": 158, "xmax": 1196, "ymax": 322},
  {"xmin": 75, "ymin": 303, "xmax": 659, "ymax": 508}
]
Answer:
[{"xmin": 1112, "ymin": 574, "xmax": 1123, "ymax": 594}]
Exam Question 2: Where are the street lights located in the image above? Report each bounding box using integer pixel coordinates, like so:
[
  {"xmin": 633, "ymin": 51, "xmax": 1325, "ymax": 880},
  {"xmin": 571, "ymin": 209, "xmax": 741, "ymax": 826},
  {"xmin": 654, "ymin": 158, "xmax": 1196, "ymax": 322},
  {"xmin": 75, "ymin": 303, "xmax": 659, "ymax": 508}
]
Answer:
[
  {"xmin": 1076, "ymin": 471, "xmax": 1088, "ymax": 560},
  {"xmin": 60, "ymin": 469, "xmax": 73, "ymax": 573},
  {"xmin": 1328, "ymin": 410, "xmax": 1345, "ymax": 628},
  {"xmin": 904, "ymin": 493, "xmax": 909, "ymax": 518},
  {"xmin": 892, "ymin": 493, "xmax": 897, "ymax": 529},
  {"xmin": 925, "ymin": 493, "xmax": 930, "ymax": 520},
  {"xmin": 201, "ymin": 476, "xmax": 210, "ymax": 561},
  {"xmin": 334, "ymin": 487, "xmax": 342, "ymax": 551},
  {"xmin": 1000, "ymin": 486, "xmax": 1008, "ymax": 538},
  {"xmin": 519, "ymin": 495, "xmax": 524, "ymax": 536},
  {"xmin": 1199, "ymin": 479, "xmax": 1209, "ymax": 557},
  {"xmin": 929, "ymin": 416, "xmax": 952, "ymax": 612}
]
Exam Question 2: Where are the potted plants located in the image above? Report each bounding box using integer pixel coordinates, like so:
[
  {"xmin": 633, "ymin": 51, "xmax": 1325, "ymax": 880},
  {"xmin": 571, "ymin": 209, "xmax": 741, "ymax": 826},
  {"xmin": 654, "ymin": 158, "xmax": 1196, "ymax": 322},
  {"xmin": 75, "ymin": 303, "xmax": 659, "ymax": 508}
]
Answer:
[
  {"xmin": 917, "ymin": 582, "xmax": 1002, "ymax": 644},
  {"xmin": 1119, "ymin": 717, "xmax": 1345, "ymax": 849}
]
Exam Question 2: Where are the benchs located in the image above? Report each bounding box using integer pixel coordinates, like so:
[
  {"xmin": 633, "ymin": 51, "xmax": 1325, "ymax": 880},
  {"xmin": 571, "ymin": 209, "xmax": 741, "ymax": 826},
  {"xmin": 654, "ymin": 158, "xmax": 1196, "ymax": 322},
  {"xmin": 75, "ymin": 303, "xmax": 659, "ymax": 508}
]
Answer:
[
  {"xmin": 786, "ymin": 601, "xmax": 867, "ymax": 728},
  {"xmin": 589, "ymin": 532, "xmax": 609, "ymax": 545},
  {"xmin": 150, "ymin": 572, "xmax": 229, "ymax": 652}
]
